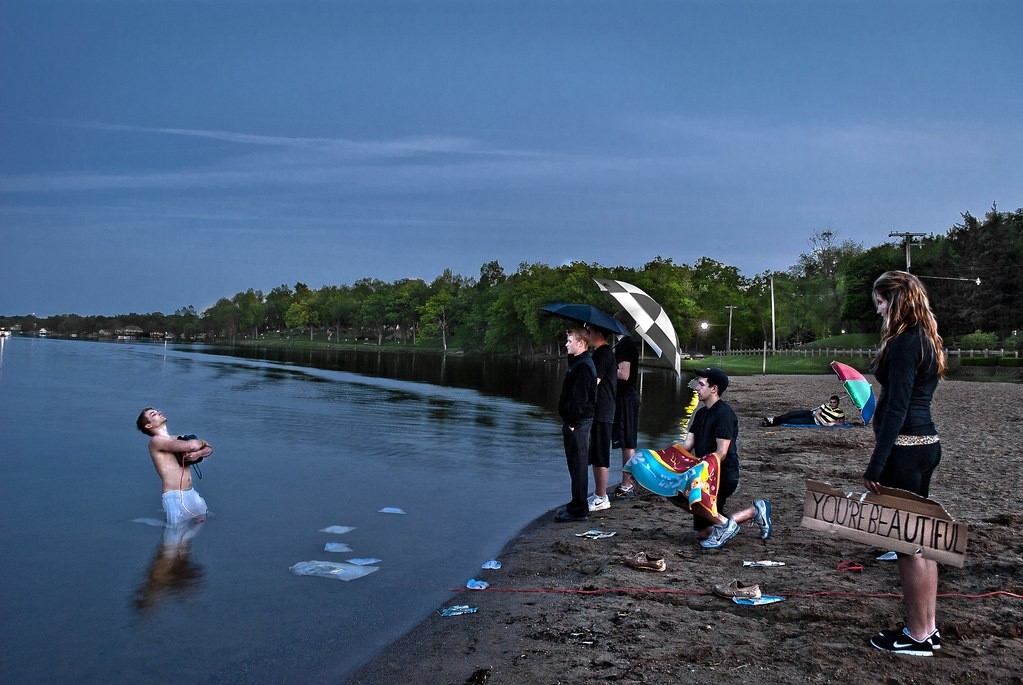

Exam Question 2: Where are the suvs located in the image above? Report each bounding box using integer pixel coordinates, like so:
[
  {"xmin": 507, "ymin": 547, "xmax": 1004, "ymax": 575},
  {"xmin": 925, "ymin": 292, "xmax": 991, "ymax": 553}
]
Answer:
[{"xmin": 680, "ymin": 353, "xmax": 691, "ymax": 359}]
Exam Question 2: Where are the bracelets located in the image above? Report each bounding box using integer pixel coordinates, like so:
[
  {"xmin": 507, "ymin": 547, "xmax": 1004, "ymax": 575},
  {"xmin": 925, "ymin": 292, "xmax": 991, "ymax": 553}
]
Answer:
[{"xmin": 200, "ymin": 439, "xmax": 206, "ymax": 448}]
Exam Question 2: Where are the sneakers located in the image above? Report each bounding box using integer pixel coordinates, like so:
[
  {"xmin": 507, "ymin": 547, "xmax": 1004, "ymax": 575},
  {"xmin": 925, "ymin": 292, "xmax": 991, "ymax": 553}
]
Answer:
[
  {"xmin": 752, "ymin": 497, "xmax": 772, "ymax": 540},
  {"xmin": 761, "ymin": 416, "xmax": 771, "ymax": 426},
  {"xmin": 698, "ymin": 518, "xmax": 740, "ymax": 549},
  {"xmin": 870, "ymin": 626, "xmax": 941, "ymax": 657},
  {"xmin": 587, "ymin": 493, "xmax": 611, "ymax": 511},
  {"xmin": 553, "ymin": 507, "xmax": 589, "ymax": 523},
  {"xmin": 608, "ymin": 482, "xmax": 635, "ymax": 500}
]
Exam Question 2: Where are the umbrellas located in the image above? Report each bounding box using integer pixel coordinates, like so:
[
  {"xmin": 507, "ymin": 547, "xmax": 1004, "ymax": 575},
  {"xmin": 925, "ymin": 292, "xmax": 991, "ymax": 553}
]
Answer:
[
  {"xmin": 538, "ymin": 303, "xmax": 632, "ymax": 336},
  {"xmin": 592, "ymin": 276, "xmax": 681, "ymax": 380},
  {"xmin": 811, "ymin": 360, "xmax": 876, "ymax": 426}
]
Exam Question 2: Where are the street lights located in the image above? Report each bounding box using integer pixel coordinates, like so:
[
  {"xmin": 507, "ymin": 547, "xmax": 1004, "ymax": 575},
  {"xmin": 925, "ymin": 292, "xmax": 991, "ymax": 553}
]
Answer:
[{"xmin": 726, "ymin": 306, "xmax": 737, "ymax": 350}]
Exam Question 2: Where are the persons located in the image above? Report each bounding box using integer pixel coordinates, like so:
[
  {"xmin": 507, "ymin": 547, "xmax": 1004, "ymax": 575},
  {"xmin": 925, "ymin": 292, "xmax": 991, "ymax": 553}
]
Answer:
[
  {"xmin": 135, "ymin": 407, "xmax": 213, "ymax": 523},
  {"xmin": 862, "ymin": 271, "xmax": 948, "ymax": 656},
  {"xmin": 680, "ymin": 367, "xmax": 772, "ymax": 549},
  {"xmin": 553, "ymin": 324, "xmax": 639, "ymax": 523},
  {"xmin": 763, "ymin": 395, "xmax": 845, "ymax": 428}
]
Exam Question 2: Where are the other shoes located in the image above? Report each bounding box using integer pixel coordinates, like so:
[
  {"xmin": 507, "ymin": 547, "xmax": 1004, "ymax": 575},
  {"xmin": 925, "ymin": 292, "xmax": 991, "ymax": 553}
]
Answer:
[
  {"xmin": 624, "ymin": 552, "xmax": 667, "ymax": 571},
  {"xmin": 711, "ymin": 579, "xmax": 762, "ymax": 599}
]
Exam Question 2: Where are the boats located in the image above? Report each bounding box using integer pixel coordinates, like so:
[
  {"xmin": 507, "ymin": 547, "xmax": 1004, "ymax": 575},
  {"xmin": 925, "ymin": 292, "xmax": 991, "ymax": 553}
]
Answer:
[
  {"xmin": 0, "ymin": 327, "xmax": 11, "ymax": 337},
  {"xmin": 39, "ymin": 328, "xmax": 48, "ymax": 336}
]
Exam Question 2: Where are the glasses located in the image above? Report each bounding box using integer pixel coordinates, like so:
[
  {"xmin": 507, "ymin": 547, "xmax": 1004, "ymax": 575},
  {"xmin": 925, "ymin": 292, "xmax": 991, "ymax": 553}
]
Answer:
[{"xmin": 830, "ymin": 401, "xmax": 837, "ymax": 403}]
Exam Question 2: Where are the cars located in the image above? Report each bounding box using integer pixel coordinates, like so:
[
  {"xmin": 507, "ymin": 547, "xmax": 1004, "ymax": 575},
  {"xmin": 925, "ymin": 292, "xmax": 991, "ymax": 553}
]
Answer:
[{"xmin": 694, "ymin": 353, "xmax": 704, "ymax": 358}]
